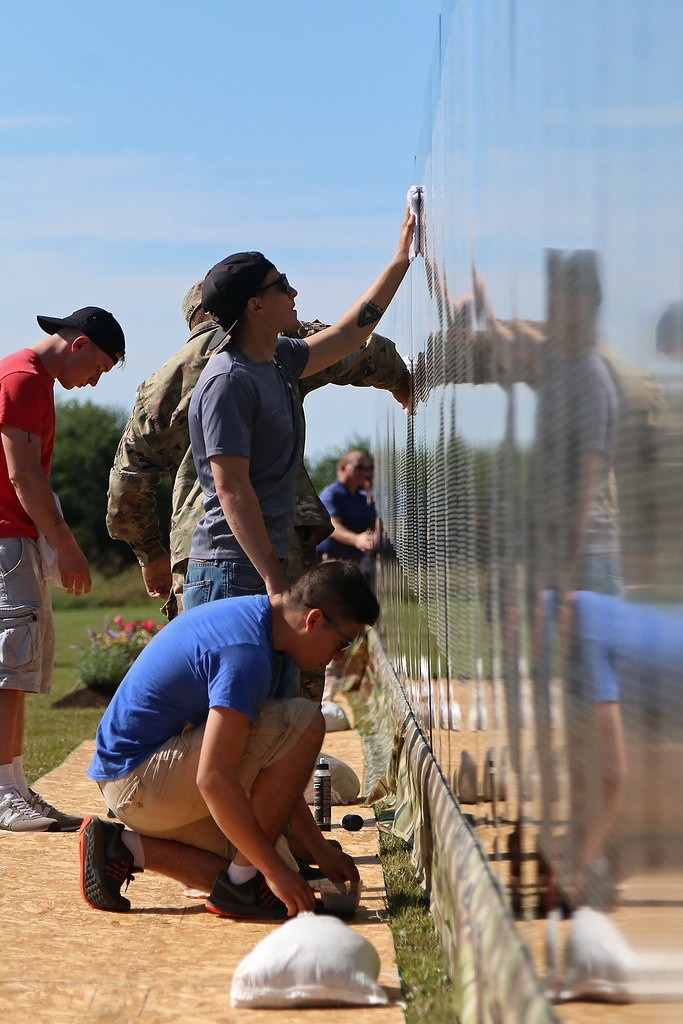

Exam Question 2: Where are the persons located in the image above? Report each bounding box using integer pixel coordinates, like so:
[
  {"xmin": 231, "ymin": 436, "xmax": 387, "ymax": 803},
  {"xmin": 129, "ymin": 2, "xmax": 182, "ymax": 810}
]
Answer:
[
  {"xmin": 0, "ymin": 305, "xmax": 126, "ymax": 834},
  {"xmin": 416, "ymin": 217, "xmax": 622, "ymax": 595},
  {"xmin": 76, "ymin": 559, "xmax": 381, "ymax": 922},
  {"xmin": 487, "ymin": 565, "xmax": 681, "ymax": 910},
  {"xmin": 316, "ymin": 448, "xmax": 376, "ymax": 561},
  {"xmin": 104, "ymin": 276, "xmax": 411, "ymax": 705},
  {"xmin": 181, "ymin": 200, "xmax": 415, "ymax": 610}
]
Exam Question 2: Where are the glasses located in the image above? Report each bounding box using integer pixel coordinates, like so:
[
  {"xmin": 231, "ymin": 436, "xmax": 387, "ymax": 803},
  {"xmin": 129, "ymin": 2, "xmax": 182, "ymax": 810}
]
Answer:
[
  {"xmin": 256, "ymin": 272, "xmax": 290, "ymax": 294},
  {"xmin": 304, "ymin": 603, "xmax": 354, "ymax": 653}
]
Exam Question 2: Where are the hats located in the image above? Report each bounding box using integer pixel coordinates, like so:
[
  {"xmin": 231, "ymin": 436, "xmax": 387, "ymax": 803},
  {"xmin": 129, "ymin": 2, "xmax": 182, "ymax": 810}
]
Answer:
[
  {"xmin": 181, "ymin": 277, "xmax": 204, "ymax": 332},
  {"xmin": 35, "ymin": 306, "xmax": 126, "ymax": 365},
  {"xmin": 202, "ymin": 252, "xmax": 276, "ymax": 351}
]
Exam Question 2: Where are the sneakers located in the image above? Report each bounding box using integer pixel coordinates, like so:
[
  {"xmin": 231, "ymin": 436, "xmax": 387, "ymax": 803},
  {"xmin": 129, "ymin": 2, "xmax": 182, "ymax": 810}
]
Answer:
[
  {"xmin": 77, "ymin": 814, "xmax": 145, "ymax": 913},
  {"xmin": 205, "ymin": 873, "xmax": 325, "ymax": 921},
  {"xmin": 0, "ymin": 788, "xmax": 83, "ymax": 833}
]
